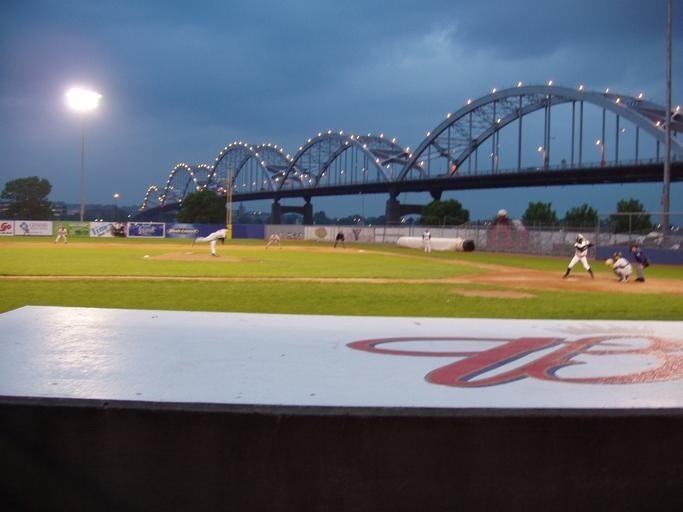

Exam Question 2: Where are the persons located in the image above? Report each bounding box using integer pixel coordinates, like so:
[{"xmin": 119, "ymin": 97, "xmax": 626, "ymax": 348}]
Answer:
[
  {"xmin": 629, "ymin": 244, "xmax": 651, "ymax": 282},
  {"xmin": 54, "ymin": 227, "xmax": 67, "ymax": 243},
  {"xmin": 561, "ymin": 234, "xmax": 595, "ymax": 279},
  {"xmin": 333, "ymin": 231, "xmax": 346, "ymax": 248},
  {"xmin": 610, "ymin": 250, "xmax": 633, "ymax": 282},
  {"xmin": 422, "ymin": 229, "xmax": 431, "ymax": 253},
  {"xmin": 264, "ymin": 231, "xmax": 280, "ymax": 248},
  {"xmin": 193, "ymin": 226, "xmax": 229, "ymax": 256}
]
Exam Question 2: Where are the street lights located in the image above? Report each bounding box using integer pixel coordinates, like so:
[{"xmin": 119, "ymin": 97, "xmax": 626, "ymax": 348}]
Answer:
[
  {"xmin": 113, "ymin": 192, "xmax": 119, "ymax": 220},
  {"xmin": 65, "ymin": 86, "xmax": 103, "ymax": 219}
]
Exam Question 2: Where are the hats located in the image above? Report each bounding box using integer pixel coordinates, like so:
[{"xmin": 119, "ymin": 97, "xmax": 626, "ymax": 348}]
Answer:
[
  {"xmin": 613, "ymin": 252, "xmax": 622, "ymax": 261},
  {"xmin": 577, "ymin": 234, "xmax": 584, "ymax": 243}
]
